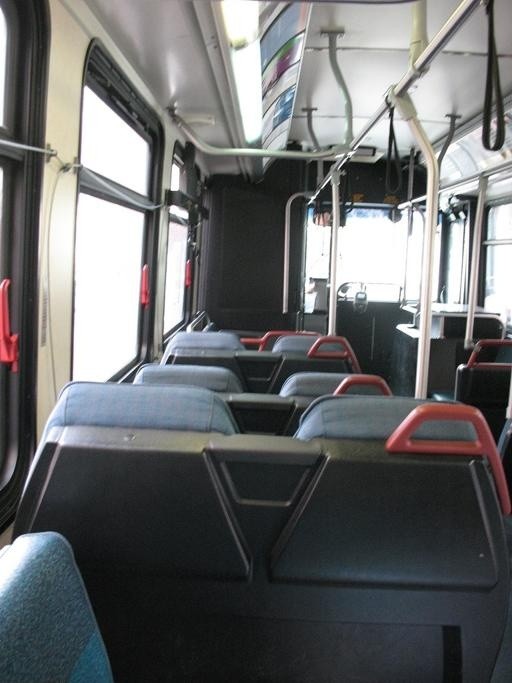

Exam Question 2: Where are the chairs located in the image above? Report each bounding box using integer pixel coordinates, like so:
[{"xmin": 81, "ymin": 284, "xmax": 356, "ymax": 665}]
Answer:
[{"xmin": 0, "ymin": 336, "xmax": 512, "ymax": 683}]
